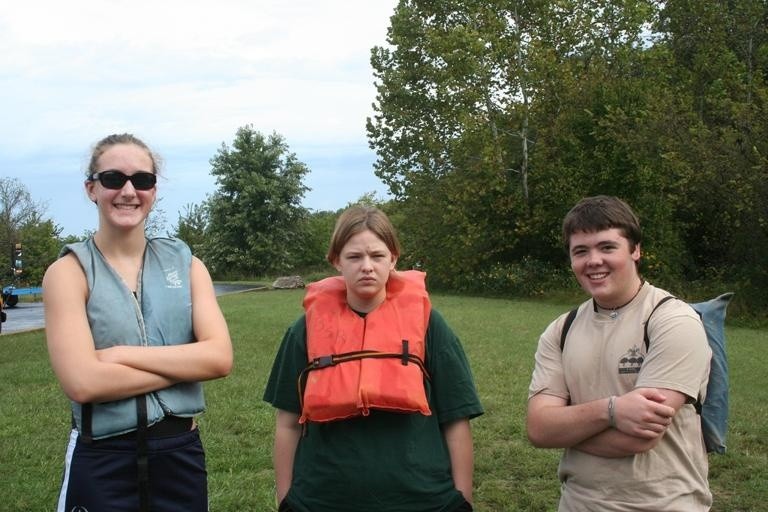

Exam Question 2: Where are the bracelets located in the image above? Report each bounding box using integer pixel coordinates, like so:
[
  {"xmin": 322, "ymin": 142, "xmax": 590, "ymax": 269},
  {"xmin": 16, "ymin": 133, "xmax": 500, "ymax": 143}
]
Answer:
[{"xmin": 606, "ymin": 394, "xmax": 620, "ymax": 434}]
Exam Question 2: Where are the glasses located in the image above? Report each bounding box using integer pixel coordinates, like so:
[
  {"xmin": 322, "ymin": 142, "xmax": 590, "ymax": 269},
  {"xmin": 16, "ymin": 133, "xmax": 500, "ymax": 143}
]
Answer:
[{"xmin": 89, "ymin": 169, "xmax": 157, "ymax": 190}]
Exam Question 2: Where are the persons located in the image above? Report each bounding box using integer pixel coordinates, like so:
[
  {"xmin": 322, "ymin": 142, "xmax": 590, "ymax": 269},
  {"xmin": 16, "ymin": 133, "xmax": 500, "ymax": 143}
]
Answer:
[
  {"xmin": 34, "ymin": 131, "xmax": 234, "ymax": 512},
  {"xmin": 520, "ymin": 192, "xmax": 716, "ymax": 512},
  {"xmin": 262, "ymin": 196, "xmax": 488, "ymax": 512}
]
